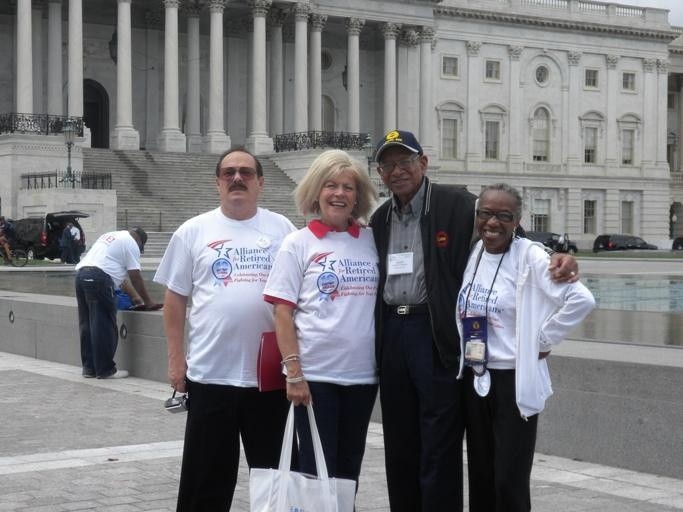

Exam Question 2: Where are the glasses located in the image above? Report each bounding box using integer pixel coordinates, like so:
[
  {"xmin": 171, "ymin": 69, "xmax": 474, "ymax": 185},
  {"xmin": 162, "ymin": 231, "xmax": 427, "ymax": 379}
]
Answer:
[
  {"xmin": 216, "ymin": 166, "xmax": 257, "ymax": 181},
  {"xmin": 376, "ymin": 158, "xmax": 420, "ymax": 177},
  {"xmin": 475, "ymin": 209, "xmax": 519, "ymax": 223}
]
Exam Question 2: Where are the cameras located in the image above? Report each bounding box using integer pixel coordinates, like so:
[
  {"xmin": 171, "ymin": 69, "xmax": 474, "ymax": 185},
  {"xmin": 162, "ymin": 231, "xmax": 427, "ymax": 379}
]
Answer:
[{"xmin": 165, "ymin": 397, "xmax": 189, "ymax": 413}]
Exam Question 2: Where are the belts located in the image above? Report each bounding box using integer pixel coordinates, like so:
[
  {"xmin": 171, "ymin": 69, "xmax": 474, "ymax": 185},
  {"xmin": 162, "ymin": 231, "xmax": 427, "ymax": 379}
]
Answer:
[
  {"xmin": 381, "ymin": 303, "xmax": 430, "ymax": 317},
  {"xmin": 79, "ymin": 266, "xmax": 111, "ymax": 278}
]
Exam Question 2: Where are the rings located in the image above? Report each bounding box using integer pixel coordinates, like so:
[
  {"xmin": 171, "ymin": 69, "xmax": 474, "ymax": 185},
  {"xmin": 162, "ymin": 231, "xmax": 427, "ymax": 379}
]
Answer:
[{"xmin": 570, "ymin": 271, "xmax": 575, "ymax": 276}]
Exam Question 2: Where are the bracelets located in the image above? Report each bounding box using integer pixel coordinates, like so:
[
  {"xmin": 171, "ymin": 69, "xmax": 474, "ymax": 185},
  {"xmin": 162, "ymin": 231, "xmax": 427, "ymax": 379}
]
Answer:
[
  {"xmin": 283, "ymin": 354, "xmax": 300, "ymax": 360},
  {"xmin": 281, "ymin": 357, "xmax": 300, "ymax": 364},
  {"xmin": 285, "ymin": 376, "xmax": 307, "ymax": 383}
]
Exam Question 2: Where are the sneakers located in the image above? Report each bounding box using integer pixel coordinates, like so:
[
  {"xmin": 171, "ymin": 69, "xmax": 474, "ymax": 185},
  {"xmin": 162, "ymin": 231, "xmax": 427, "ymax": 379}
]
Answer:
[{"xmin": 82, "ymin": 369, "xmax": 129, "ymax": 379}]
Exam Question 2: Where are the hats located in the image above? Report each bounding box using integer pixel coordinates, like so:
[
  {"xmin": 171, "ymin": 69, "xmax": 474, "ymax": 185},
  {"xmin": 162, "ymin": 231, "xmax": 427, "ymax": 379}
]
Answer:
[
  {"xmin": 131, "ymin": 225, "xmax": 148, "ymax": 254},
  {"xmin": 373, "ymin": 129, "xmax": 423, "ymax": 159}
]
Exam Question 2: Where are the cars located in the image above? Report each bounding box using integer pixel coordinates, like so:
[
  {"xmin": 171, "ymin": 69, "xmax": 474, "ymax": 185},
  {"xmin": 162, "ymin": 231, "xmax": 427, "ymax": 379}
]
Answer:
[{"xmin": 671, "ymin": 236, "xmax": 683, "ymax": 251}]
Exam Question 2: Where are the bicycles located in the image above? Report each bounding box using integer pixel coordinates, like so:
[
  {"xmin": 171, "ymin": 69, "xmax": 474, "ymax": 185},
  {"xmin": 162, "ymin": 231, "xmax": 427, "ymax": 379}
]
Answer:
[{"xmin": 0, "ymin": 240, "xmax": 29, "ymax": 268}]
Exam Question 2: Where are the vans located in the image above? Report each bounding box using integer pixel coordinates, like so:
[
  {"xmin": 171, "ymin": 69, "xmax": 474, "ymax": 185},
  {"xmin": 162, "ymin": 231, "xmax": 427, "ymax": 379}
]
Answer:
[
  {"xmin": 591, "ymin": 232, "xmax": 658, "ymax": 257},
  {"xmin": 2, "ymin": 210, "xmax": 87, "ymax": 261},
  {"xmin": 524, "ymin": 230, "xmax": 578, "ymax": 256}
]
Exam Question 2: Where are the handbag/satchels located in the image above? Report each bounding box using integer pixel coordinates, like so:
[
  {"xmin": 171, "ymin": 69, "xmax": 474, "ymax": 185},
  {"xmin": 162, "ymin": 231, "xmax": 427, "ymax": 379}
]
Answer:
[{"xmin": 247, "ymin": 460, "xmax": 357, "ymax": 511}]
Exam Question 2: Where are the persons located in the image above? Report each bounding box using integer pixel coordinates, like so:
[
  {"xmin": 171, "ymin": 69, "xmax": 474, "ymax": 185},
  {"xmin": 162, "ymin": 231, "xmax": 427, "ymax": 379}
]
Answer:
[
  {"xmin": 153, "ymin": 146, "xmax": 300, "ymax": 512},
  {"xmin": 0, "ymin": 215, "xmax": 18, "ymax": 265},
  {"xmin": 59, "ymin": 224, "xmax": 77, "ymax": 263},
  {"xmin": 455, "ymin": 182, "xmax": 596, "ymax": 512},
  {"xmin": 75, "ymin": 226, "xmax": 155, "ymax": 379},
  {"xmin": 555, "ymin": 234, "xmax": 565, "ymax": 252},
  {"xmin": 69, "ymin": 223, "xmax": 82, "ymax": 262},
  {"xmin": 368, "ymin": 130, "xmax": 579, "ymax": 512},
  {"xmin": 263, "ymin": 148, "xmax": 379, "ymax": 512}
]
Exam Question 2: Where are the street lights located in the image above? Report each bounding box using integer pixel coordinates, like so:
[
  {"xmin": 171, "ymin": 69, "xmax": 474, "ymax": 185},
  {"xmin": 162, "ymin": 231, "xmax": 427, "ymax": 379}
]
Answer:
[
  {"xmin": 361, "ymin": 132, "xmax": 375, "ymax": 180},
  {"xmin": 58, "ymin": 118, "xmax": 81, "ymax": 188}
]
